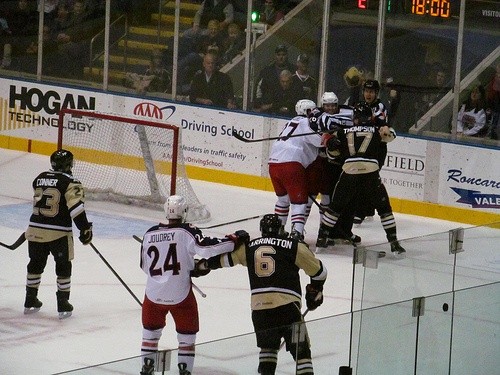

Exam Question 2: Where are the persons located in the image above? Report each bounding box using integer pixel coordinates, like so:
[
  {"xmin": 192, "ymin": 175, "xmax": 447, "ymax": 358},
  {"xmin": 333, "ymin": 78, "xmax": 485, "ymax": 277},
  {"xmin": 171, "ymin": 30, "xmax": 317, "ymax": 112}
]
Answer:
[
  {"xmin": 1, "ymin": 0, "xmax": 499, "ymax": 141},
  {"xmin": 352, "ymin": 78, "xmax": 390, "ymax": 128},
  {"xmin": 312, "ymin": 92, "xmax": 359, "ymax": 243},
  {"xmin": 319, "ymin": 102, "xmax": 406, "ymax": 257},
  {"xmin": 22, "ymin": 150, "xmax": 93, "ymax": 312},
  {"xmin": 266, "ymin": 97, "xmax": 324, "ymax": 244},
  {"xmin": 197, "ymin": 213, "xmax": 329, "ymax": 375},
  {"xmin": 137, "ymin": 195, "xmax": 250, "ymax": 375}
]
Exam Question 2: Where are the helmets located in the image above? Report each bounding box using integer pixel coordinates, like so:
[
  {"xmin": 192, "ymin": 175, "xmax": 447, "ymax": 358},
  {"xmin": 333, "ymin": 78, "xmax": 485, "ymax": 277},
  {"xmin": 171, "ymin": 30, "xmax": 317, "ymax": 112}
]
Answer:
[
  {"xmin": 361, "ymin": 80, "xmax": 380, "ymax": 97},
  {"xmin": 295, "ymin": 99, "xmax": 316, "ymax": 117},
  {"xmin": 49, "ymin": 150, "xmax": 74, "ymax": 173},
  {"xmin": 320, "ymin": 91, "xmax": 338, "ymax": 112},
  {"xmin": 260, "ymin": 214, "xmax": 282, "ymax": 237},
  {"xmin": 161, "ymin": 194, "xmax": 188, "ymax": 220},
  {"xmin": 353, "ymin": 102, "xmax": 372, "ymax": 126}
]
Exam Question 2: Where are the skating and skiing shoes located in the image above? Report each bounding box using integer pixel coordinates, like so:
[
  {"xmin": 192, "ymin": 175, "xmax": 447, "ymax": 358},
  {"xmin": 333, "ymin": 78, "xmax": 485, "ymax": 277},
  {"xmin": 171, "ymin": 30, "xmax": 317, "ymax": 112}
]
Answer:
[
  {"xmin": 315, "ymin": 231, "xmax": 334, "ymax": 253},
  {"xmin": 277, "ymin": 225, "xmax": 289, "ymax": 239},
  {"xmin": 291, "ymin": 229, "xmax": 310, "ymax": 249},
  {"xmin": 336, "ymin": 231, "xmax": 361, "ymax": 247},
  {"xmin": 389, "ymin": 236, "xmax": 405, "ymax": 257},
  {"xmin": 56, "ymin": 291, "xmax": 74, "ymax": 320},
  {"xmin": 23, "ymin": 285, "xmax": 44, "ymax": 315}
]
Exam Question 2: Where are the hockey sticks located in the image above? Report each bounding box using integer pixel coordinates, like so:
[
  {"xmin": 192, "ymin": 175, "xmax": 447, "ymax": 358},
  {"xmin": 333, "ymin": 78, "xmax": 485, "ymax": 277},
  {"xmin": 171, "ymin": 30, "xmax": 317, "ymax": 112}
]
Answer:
[
  {"xmin": 89, "ymin": 242, "xmax": 143, "ymax": 308},
  {"xmin": 191, "ymin": 214, "xmax": 262, "ymax": 229},
  {"xmin": 307, "ymin": 191, "xmax": 387, "ymax": 259},
  {"xmin": 232, "ymin": 130, "xmax": 317, "ymax": 143},
  {"xmin": 0, "ymin": 232, "xmax": 27, "ymax": 251},
  {"xmin": 277, "ymin": 308, "xmax": 309, "ymax": 352},
  {"xmin": 132, "ymin": 233, "xmax": 206, "ymax": 298}
]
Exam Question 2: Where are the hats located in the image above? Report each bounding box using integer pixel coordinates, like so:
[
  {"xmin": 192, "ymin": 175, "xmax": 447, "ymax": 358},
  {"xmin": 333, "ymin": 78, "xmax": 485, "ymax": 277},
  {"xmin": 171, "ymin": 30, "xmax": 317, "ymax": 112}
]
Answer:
[
  {"xmin": 274, "ymin": 44, "xmax": 288, "ymax": 54},
  {"xmin": 296, "ymin": 54, "xmax": 310, "ymax": 65}
]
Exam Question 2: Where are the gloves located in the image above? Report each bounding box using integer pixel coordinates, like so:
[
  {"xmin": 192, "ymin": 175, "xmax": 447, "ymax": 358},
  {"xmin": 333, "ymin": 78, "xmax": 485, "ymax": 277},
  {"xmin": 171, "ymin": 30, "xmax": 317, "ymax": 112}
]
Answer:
[
  {"xmin": 227, "ymin": 229, "xmax": 250, "ymax": 250},
  {"xmin": 78, "ymin": 222, "xmax": 93, "ymax": 246},
  {"xmin": 190, "ymin": 258, "xmax": 210, "ymax": 278},
  {"xmin": 304, "ymin": 284, "xmax": 325, "ymax": 310}
]
